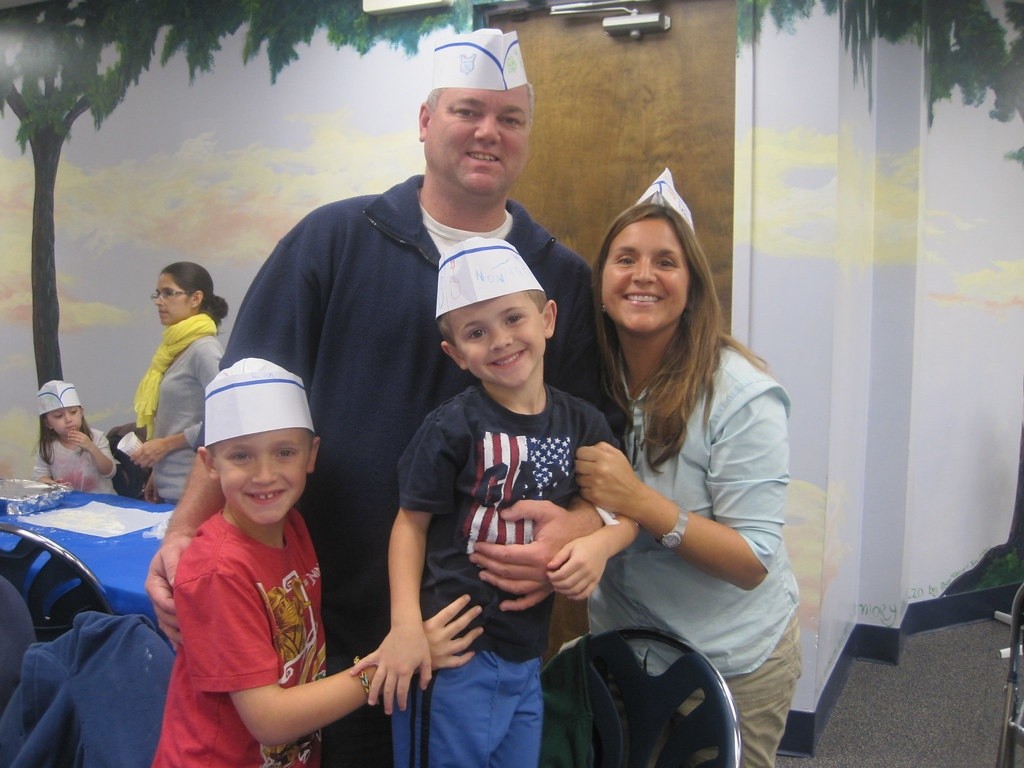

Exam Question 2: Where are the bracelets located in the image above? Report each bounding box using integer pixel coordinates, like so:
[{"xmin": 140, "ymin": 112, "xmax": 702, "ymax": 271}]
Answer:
[{"xmin": 353, "ymin": 655, "xmax": 380, "ymax": 705}]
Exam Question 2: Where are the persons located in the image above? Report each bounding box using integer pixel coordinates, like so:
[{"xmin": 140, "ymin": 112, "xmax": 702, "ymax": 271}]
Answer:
[
  {"xmin": 148, "ymin": 28, "xmax": 604, "ymax": 768},
  {"xmin": 348, "ymin": 236, "xmax": 640, "ymax": 768},
  {"xmin": 32, "ymin": 380, "xmax": 118, "ymax": 495},
  {"xmin": 151, "ymin": 358, "xmax": 483, "ymax": 768},
  {"xmin": 574, "ymin": 167, "xmax": 801, "ymax": 768},
  {"xmin": 130, "ymin": 261, "xmax": 224, "ymax": 505}
]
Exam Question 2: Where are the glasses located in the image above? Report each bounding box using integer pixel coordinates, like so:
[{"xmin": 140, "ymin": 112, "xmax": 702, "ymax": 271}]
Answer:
[{"xmin": 150, "ymin": 285, "xmax": 190, "ymax": 303}]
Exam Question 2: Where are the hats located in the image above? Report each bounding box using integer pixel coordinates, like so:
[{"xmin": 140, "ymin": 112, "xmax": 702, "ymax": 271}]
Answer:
[
  {"xmin": 634, "ymin": 166, "xmax": 695, "ymax": 235},
  {"xmin": 37, "ymin": 380, "xmax": 82, "ymax": 416},
  {"xmin": 203, "ymin": 358, "xmax": 314, "ymax": 448},
  {"xmin": 431, "ymin": 29, "xmax": 526, "ymax": 91},
  {"xmin": 435, "ymin": 237, "xmax": 544, "ymax": 318}
]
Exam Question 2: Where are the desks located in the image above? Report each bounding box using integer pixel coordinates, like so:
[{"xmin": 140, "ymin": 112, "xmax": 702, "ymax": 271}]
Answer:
[{"xmin": 0, "ymin": 490, "xmax": 178, "ymax": 626}]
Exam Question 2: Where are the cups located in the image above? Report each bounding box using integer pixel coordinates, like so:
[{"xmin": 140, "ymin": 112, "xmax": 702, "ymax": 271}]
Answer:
[{"xmin": 117, "ymin": 431, "xmax": 154, "ymax": 468}]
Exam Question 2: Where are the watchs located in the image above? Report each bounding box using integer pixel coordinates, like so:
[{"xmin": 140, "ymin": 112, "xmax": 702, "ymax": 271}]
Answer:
[{"xmin": 654, "ymin": 506, "xmax": 690, "ymax": 550}]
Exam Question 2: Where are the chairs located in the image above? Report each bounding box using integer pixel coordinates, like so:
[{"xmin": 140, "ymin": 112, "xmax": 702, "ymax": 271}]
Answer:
[
  {"xmin": 538, "ymin": 625, "xmax": 743, "ymax": 768},
  {"xmin": 0, "ymin": 521, "xmax": 114, "ymax": 713}
]
